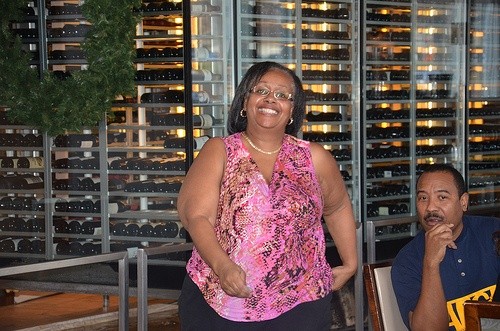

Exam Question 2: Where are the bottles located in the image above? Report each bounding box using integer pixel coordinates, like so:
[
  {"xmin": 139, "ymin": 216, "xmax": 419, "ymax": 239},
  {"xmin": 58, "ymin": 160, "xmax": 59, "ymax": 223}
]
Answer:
[
  {"xmin": 0, "ymin": 0, "xmax": 224, "ymax": 260},
  {"xmin": 238, "ymin": 4, "xmax": 500, "ymax": 237}
]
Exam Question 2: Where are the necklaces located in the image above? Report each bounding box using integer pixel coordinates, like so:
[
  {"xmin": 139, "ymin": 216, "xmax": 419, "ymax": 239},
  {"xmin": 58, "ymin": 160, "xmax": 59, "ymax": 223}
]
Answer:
[{"xmin": 242, "ymin": 132, "xmax": 281, "ymax": 154}]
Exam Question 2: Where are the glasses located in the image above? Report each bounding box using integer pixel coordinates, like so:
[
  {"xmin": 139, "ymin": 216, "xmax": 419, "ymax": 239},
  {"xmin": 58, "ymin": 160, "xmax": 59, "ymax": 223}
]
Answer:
[{"xmin": 250, "ymin": 86, "xmax": 293, "ymax": 100}]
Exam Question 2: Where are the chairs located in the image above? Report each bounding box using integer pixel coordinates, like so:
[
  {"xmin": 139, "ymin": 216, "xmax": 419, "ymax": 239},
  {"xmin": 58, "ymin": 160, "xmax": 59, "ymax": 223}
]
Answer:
[
  {"xmin": 361, "ymin": 261, "xmax": 408, "ymax": 331},
  {"xmin": 463, "ymin": 300, "xmax": 500, "ymax": 331}
]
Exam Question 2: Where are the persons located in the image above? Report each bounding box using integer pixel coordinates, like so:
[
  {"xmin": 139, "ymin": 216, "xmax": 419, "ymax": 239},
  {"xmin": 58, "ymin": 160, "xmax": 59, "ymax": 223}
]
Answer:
[
  {"xmin": 177, "ymin": 60, "xmax": 358, "ymax": 331},
  {"xmin": 391, "ymin": 164, "xmax": 500, "ymax": 331}
]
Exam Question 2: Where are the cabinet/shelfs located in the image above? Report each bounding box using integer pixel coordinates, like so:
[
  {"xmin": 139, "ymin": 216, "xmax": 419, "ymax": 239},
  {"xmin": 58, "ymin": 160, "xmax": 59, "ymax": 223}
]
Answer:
[{"xmin": 0, "ymin": 0, "xmax": 500, "ymax": 268}]
